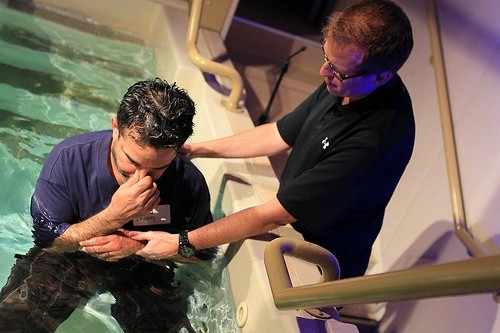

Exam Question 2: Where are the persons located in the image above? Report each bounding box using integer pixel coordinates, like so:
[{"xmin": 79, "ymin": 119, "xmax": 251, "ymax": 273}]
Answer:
[
  {"xmin": 128, "ymin": 0, "xmax": 416, "ymax": 315},
  {"xmin": 0, "ymin": 77, "xmax": 214, "ymax": 333}
]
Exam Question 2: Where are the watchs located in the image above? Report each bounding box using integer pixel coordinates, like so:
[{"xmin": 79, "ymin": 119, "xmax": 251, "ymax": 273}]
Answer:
[{"xmin": 178, "ymin": 230, "xmax": 196, "ymax": 258}]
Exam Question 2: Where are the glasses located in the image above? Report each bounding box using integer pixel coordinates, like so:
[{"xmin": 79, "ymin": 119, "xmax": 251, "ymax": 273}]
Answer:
[{"xmin": 320, "ymin": 39, "xmax": 382, "ymax": 82}]
